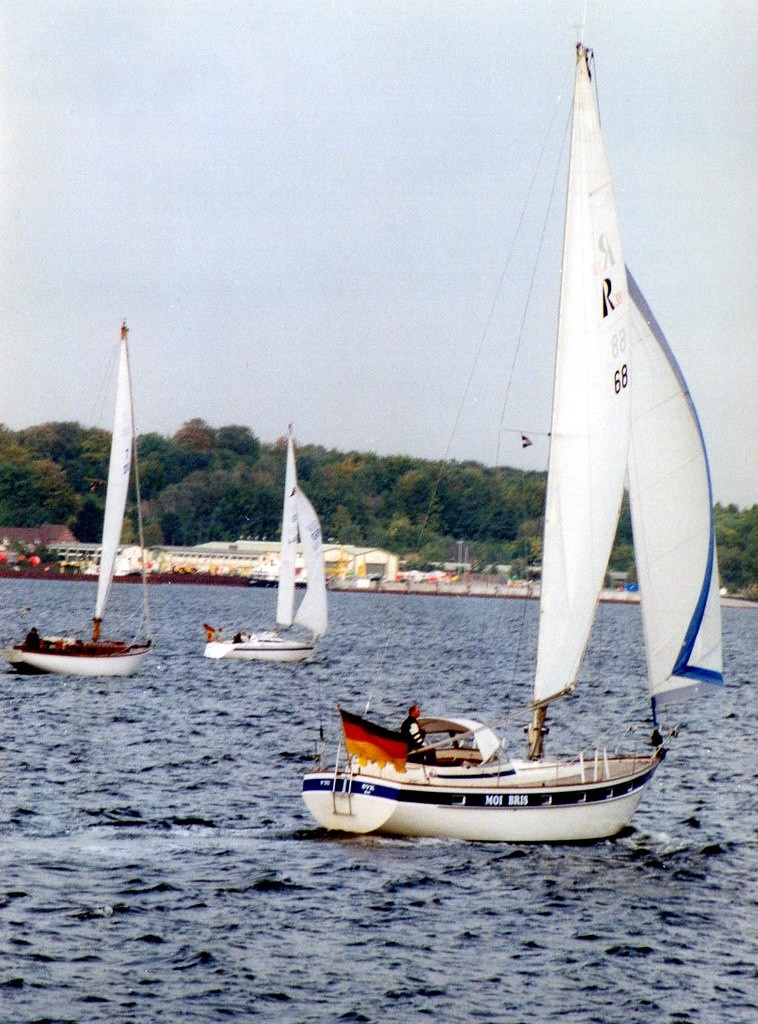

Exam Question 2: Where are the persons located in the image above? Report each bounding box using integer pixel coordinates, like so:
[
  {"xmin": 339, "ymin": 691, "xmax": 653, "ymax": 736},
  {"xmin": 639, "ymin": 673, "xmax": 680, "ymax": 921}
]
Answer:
[
  {"xmin": 25, "ymin": 627, "xmax": 40, "ymax": 648},
  {"xmin": 232, "ymin": 630, "xmax": 253, "ymax": 643},
  {"xmin": 401, "ymin": 703, "xmax": 436, "ymax": 765},
  {"xmin": 210, "ymin": 627, "xmax": 226, "ymax": 643}
]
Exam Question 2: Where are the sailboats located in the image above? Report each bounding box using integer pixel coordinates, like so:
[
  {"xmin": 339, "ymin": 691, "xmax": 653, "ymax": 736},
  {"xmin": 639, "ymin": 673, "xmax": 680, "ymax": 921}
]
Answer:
[
  {"xmin": 303, "ymin": 0, "xmax": 723, "ymax": 842},
  {"xmin": 203, "ymin": 423, "xmax": 327, "ymax": 662},
  {"xmin": 1, "ymin": 317, "xmax": 154, "ymax": 677}
]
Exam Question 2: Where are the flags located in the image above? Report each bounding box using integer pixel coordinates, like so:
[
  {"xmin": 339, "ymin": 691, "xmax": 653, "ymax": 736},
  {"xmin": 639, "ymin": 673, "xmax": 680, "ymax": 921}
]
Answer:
[{"xmin": 339, "ymin": 709, "xmax": 408, "ymax": 774}]
